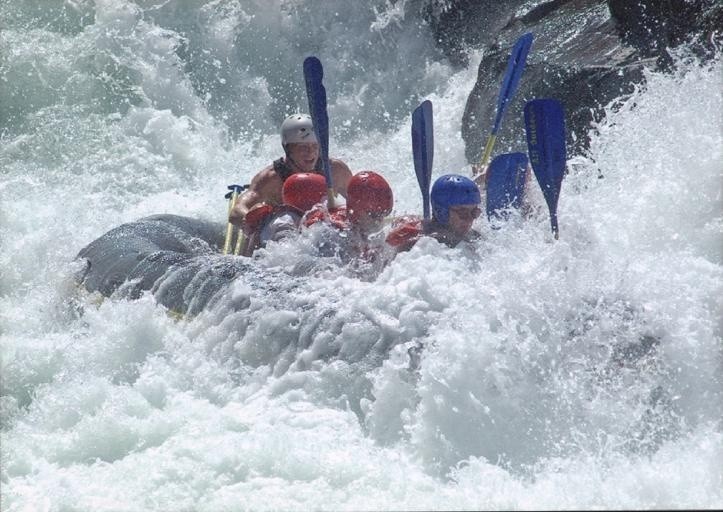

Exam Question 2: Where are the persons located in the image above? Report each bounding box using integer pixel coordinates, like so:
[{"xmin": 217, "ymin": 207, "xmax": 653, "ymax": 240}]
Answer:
[
  {"xmin": 227, "ymin": 114, "xmax": 352, "ymax": 237},
  {"xmin": 388, "ymin": 175, "xmax": 484, "ymax": 260},
  {"xmin": 309, "ymin": 170, "xmax": 393, "ymax": 263},
  {"xmin": 246, "ymin": 171, "xmax": 328, "ymax": 257}
]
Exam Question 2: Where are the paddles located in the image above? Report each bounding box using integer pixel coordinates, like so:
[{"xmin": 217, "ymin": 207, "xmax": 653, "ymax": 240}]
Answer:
[
  {"xmin": 303, "ymin": 57, "xmax": 335, "ymax": 212},
  {"xmin": 412, "ymin": 99, "xmax": 433, "ymax": 222},
  {"xmin": 526, "ymin": 100, "xmax": 566, "ymax": 241},
  {"xmin": 480, "ymin": 34, "xmax": 532, "ymax": 176},
  {"xmin": 485, "ymin": 152, "xmax": 528, "ymax": 229}
]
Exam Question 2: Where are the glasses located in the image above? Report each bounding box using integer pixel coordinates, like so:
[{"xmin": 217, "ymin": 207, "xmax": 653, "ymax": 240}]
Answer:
[{"xmin": 435, "ymin": 201, "xmax": 482, "ymax": 220}]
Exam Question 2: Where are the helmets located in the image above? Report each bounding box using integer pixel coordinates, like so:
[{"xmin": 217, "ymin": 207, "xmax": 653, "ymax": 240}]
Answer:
[
  {"xmin": 282, "ymin": 173, "xmax": 327, "ymax": 209},
  {"xmin": 430, "ymin": 174, "xmax": 481, "ymax": 227},
  {"xmin": 348, "ymin": 170, "xmax": 394, "ymax": 218},
  {"xmin": 280, "ymin": 113, "xmax": 315, "ymax": 143}
]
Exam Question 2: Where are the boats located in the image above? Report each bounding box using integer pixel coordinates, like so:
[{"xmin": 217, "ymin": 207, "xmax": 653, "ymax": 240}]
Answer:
[{"xmin": 75, "ymin": 214, "xmax": 662, "ymax": 377}]
